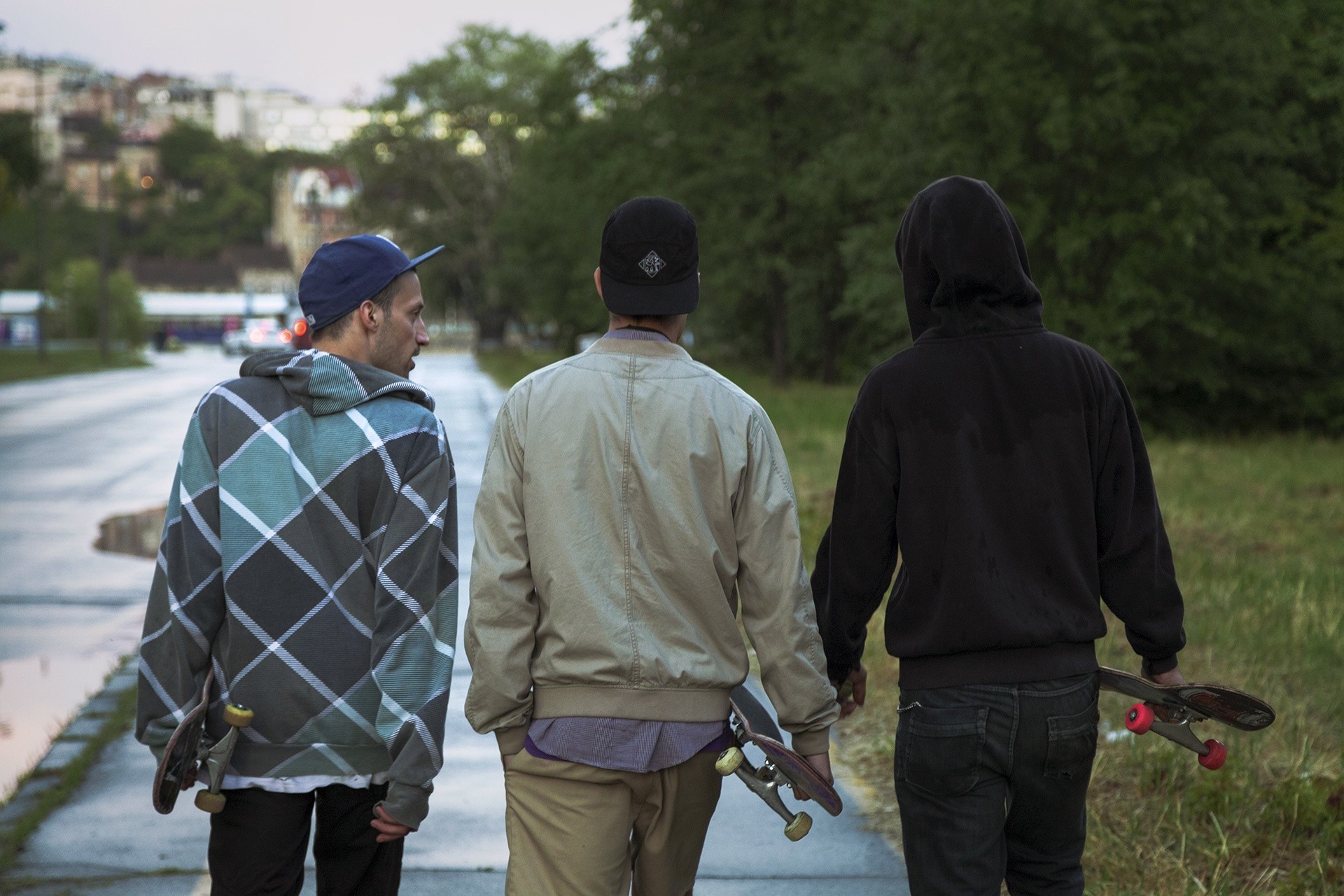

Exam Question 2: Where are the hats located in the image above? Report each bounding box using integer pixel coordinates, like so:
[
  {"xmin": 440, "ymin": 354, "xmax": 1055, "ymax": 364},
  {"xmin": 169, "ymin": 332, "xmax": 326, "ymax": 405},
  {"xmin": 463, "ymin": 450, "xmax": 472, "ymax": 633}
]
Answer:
[
  {"xmin": 600, "ymin": 197, "xmax": 700, "ymax": 315},
  {"xmin": 298, "ymin": 235, "xmax": 446, "ymax": 331}
]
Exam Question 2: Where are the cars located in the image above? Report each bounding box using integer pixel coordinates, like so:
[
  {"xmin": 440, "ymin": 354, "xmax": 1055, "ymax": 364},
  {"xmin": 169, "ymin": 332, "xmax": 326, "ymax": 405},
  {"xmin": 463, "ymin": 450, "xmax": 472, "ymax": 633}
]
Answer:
[{"xmin": 221, "ymin": 316, "xmax": 291, "ymax": 357}]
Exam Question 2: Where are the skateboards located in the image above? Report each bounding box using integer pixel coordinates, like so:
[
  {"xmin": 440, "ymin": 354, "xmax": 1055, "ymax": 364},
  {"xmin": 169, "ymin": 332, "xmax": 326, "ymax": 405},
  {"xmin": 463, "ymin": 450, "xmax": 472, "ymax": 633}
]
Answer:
[
  {"xmin": 713, "ymin": 684, "xmax": 844, "ymax": 844},
  {"xmin": 1096, "ymin": 662, "xmax": 1277, "ymax": 770},
  {"xmin": 151, "ymin": 663, "xmax": 256, "ymax": 817}
]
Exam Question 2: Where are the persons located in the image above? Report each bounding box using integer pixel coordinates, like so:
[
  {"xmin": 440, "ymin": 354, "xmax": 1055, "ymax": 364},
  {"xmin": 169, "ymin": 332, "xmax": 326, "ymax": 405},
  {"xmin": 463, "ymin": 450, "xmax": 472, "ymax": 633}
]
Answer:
[
  {"xmin": 810, "ymin": 176, "xmax": 1185, "ymax": 896},
  {"xmin": 133, "ymin": 236, "xmax": 460, "ymax": 895},
  {"xmin": 460, "ymin": 198, "xmax": 841, "ymax": 896}
]
